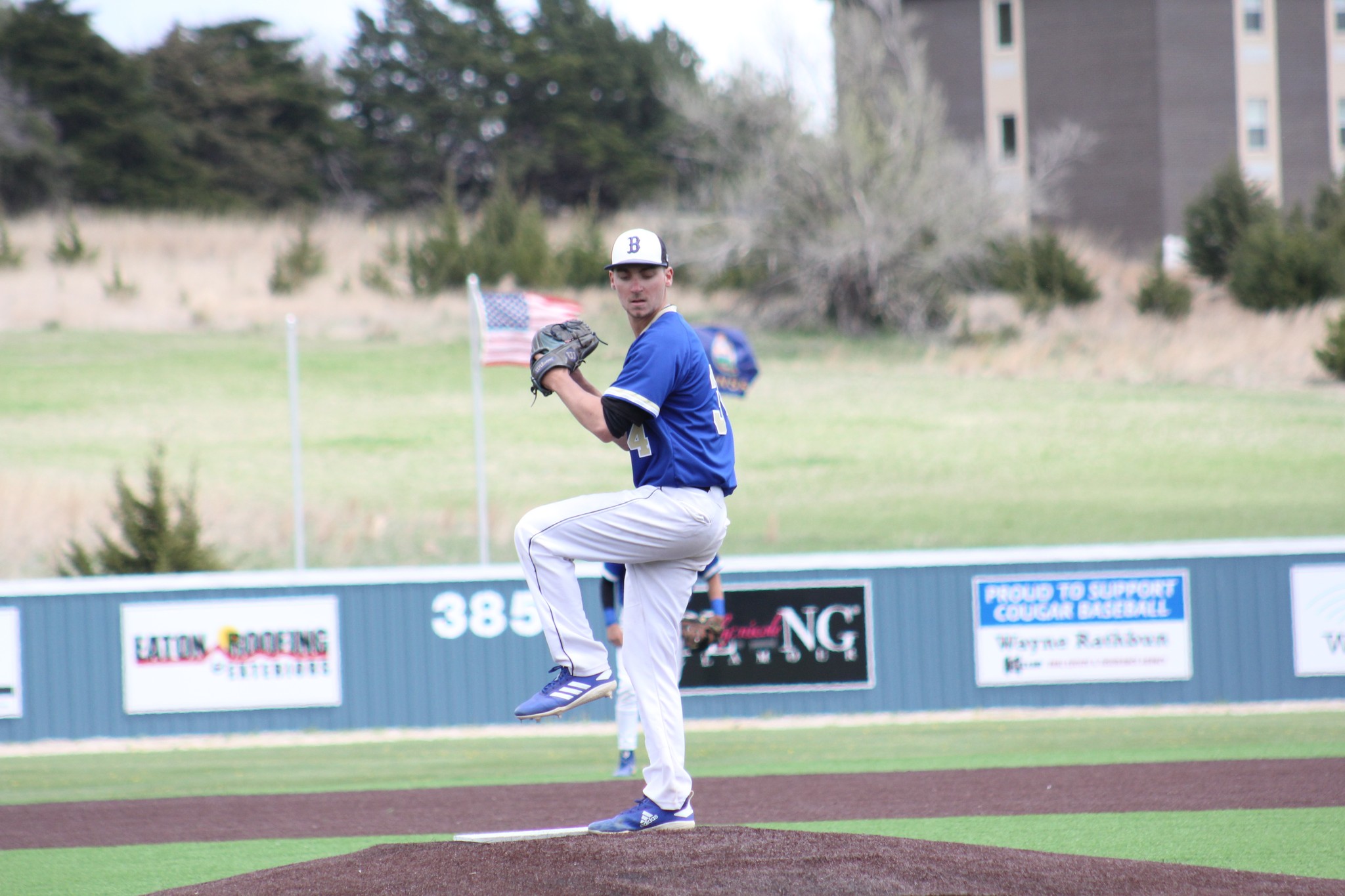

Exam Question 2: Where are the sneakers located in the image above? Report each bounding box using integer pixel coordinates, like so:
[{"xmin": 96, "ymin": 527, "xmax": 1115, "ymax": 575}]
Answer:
[
  {"xmin": 587, "ymin": 795, "xmax": 697, "ymax": 834},
  {"xmin": 514, "ymin": 665, "xmax": 617, "ymax": 722}
]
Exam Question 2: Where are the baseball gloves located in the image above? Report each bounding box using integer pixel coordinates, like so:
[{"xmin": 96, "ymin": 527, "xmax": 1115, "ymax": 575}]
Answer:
[
  {"xmin": 529, "ymin": 319, "xmax": 599, "ymax": 397},
  {"xmin": 683, "ymin": 616, "xmax": 725, "ymax": 656}
]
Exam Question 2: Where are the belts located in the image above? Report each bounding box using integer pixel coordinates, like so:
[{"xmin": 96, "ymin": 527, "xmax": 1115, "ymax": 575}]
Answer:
[{"xmin": 695, "ymin": 486, "xmax": 710, "ymax": 493}]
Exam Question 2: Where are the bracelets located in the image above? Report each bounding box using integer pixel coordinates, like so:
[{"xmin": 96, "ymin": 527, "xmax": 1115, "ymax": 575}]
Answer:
[{"xmin": 710, "ymin": 600, "xmax": 725, "ymax": 615}]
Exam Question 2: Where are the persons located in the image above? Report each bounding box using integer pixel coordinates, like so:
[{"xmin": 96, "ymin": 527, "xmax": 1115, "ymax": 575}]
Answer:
[
  {"xmin": 598, "ymin": 553, "xmax": 726, "ymax": 779},
  {"xmin": 513, "ymin": 227, "xmax": 737, "ymax": 835}
]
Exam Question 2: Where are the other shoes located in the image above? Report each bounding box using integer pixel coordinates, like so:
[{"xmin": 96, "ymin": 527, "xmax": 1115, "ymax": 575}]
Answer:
[{"xmin": 614, "ymin": 751, "xmax": 636, "ymax": 777}]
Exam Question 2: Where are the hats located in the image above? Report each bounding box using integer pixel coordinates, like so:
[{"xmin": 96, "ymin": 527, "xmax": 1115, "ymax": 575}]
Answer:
[{"xmin": 604, "ymin": 228, "xmax": 669, "ymax": 270}]
